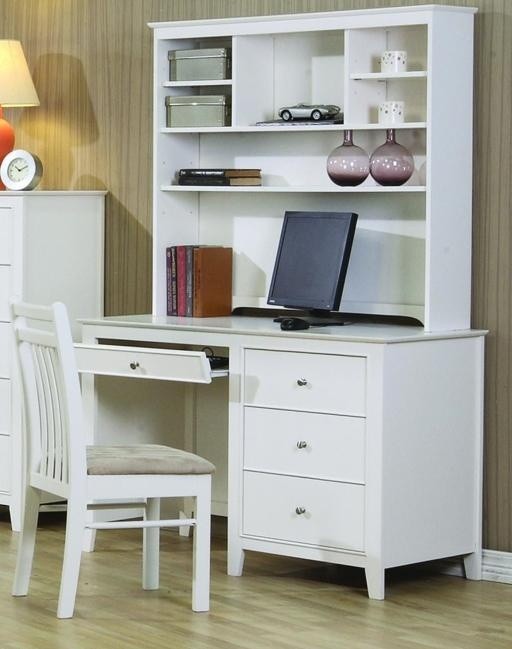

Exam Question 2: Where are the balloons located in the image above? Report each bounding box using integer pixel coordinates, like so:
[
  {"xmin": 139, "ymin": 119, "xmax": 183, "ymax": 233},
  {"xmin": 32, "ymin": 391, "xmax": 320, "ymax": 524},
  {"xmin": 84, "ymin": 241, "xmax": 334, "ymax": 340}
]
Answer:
[{"xmin": 0, "ymin": 150, "xmax": 43, "ymax": 191}]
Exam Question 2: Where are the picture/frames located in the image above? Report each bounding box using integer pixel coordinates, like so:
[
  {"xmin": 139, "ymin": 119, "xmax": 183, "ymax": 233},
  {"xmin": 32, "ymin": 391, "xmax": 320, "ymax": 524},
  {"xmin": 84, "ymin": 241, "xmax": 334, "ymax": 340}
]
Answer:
[{"xmin": 77, "ymin": 315, "xmax": 489, "ymax": 600}]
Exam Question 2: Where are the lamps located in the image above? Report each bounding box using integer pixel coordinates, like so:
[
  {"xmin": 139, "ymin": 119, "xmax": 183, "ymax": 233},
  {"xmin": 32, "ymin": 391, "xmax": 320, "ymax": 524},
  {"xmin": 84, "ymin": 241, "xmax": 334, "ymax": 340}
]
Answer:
[{"xmin": 10, "ymin": 303, "xmax": 215, "ymax": 618}]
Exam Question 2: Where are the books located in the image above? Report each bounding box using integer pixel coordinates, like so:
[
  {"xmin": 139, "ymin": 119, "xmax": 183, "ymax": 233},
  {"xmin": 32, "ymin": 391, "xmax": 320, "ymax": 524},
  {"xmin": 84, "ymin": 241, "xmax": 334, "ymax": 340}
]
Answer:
[
  {"xmin": 168, "ymin": 48, "xmax": 228, "ymax": 81},
  {"xmin": 165, "ymin": 93, "xmax": 231, "ymax": 127}
]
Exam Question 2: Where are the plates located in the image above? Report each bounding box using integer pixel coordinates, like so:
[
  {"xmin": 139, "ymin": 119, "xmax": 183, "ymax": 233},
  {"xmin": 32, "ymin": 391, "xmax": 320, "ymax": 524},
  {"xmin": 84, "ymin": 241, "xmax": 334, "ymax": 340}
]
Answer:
[
  {"xmin": 145, "ymin": 4, "xmax": 481, "ymax": 331},
  {"xmin": 1, "ymin": 186, "xmax": 111, "ymax": 530}
]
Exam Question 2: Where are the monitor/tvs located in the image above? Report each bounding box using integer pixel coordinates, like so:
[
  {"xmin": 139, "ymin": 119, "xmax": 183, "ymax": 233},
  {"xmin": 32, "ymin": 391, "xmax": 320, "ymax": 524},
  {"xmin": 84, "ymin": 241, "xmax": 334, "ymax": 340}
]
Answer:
[
  {"xmin": 192, "ymin": 247, "xmax": 233, "ymax": 317},
  {"xmin": 185, "ymin": 246, "xmax": 193, "ymax": 317},
  {"xmin": 178, "ymin": 168, "xmax": 261, "ymax": 177},
  {"xmin": 176, "ymin": 245, "xmax": 186, "ymax": 316},
  {"xmin": 172, "ymin": 245, "xmax": 177, "ymax": 316},
  {"xmin": 166, "ymin": 247, "xmax": 173, "ymax": 317},
  {"xmin": 178, "ymin": 175, "xmax": 262, "ymax": 187}
]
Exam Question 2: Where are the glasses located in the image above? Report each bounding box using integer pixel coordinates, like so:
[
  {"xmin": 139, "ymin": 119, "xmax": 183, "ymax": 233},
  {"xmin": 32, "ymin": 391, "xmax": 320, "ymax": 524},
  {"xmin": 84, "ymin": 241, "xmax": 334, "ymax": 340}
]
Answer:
[{"xmin": 0, "ymin": 38, "xmax": 42, "ymax": 189}]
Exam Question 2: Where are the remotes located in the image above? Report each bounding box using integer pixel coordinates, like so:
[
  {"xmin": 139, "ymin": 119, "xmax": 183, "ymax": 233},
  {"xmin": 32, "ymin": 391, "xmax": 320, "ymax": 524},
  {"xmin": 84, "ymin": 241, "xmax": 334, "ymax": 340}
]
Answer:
[{"xmin": 281, "ymin": 319, "xmax": 309, "ymax": 330}]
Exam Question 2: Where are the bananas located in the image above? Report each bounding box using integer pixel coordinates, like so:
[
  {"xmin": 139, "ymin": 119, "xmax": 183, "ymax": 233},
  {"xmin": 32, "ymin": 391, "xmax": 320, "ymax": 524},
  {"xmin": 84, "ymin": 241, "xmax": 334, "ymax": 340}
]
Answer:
[{"xmin": 206, "ymin": 356, "xmax": 229, "ymax": 369}]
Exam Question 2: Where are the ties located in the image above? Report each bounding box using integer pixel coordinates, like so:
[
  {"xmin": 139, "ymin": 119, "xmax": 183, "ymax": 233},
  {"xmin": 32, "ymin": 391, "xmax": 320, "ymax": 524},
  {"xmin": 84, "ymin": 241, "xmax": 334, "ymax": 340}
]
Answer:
[{"xmin": 267, "ymin": 211, "xmax": 358, "ymax": 326}]
